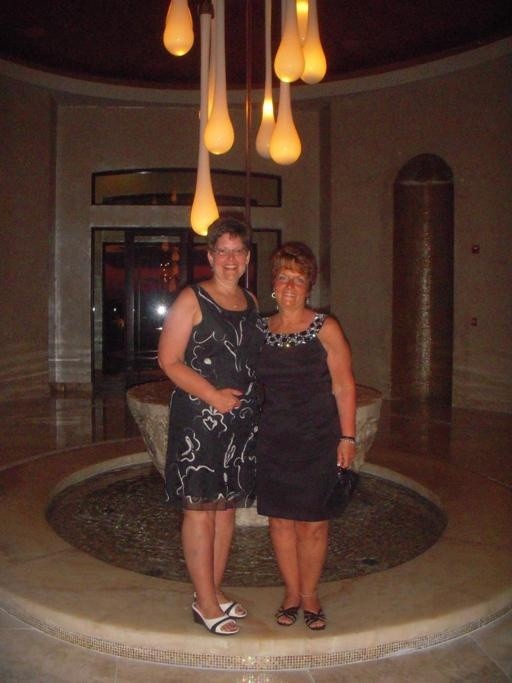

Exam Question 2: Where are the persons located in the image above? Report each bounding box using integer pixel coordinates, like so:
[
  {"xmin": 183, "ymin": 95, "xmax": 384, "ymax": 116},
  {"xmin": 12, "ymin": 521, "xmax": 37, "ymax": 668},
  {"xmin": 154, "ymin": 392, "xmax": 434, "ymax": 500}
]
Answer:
[
  {"xmin": 249, "ymin": 237, "xmax": 358, "ymax": 633},
  {"xmin": 156, "ymin": 214, "xmax": 261, "ymax": 637}
]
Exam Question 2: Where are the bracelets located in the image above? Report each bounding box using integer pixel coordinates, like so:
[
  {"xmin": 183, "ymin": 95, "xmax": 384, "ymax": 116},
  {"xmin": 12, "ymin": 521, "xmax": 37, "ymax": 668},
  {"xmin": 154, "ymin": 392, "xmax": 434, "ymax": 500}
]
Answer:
[{"xmin": 339, "ymin": 435, "xmax": 356, "ymax": 444}]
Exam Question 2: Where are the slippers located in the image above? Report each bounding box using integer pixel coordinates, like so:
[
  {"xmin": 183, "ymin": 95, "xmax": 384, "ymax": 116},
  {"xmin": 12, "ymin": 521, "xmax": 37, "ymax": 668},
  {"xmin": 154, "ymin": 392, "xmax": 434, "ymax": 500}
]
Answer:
[
  {"xmin": 276, "ymin": 602, "xmax": 301, "ymax": 625},
  {"xmin": 303, "ymin": 606, "xmax": 326, "ymax": 630}
]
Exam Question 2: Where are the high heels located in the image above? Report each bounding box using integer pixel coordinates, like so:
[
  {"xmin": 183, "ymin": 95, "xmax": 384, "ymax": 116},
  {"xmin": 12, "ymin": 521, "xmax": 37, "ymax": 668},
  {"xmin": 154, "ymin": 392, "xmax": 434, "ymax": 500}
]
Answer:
[{"xmin": 191, "ymin": 592, "xmax": 248, "ymax": 635}]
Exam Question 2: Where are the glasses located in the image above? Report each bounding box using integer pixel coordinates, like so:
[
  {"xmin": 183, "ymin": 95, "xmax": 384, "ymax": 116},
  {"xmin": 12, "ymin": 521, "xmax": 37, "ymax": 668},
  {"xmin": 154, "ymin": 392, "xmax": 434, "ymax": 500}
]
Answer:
[{"xmin": 211, "ymin": 247, "xmax": 248, "ymax": 255}]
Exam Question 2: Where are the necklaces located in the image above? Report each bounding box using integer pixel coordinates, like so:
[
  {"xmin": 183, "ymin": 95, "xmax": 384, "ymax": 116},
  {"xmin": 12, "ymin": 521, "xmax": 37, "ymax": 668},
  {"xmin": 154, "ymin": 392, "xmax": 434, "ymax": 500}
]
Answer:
[{"xmin": 229, "ymin": 302, "xmax": 240, "ymax": 309}]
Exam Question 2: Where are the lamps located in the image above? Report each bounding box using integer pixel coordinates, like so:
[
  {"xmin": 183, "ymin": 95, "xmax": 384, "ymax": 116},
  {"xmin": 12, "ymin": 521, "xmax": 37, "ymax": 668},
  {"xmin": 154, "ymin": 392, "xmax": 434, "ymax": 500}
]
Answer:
[{"xmin": 155, "ymin": 0, "xmax": 328, "ymax": 238}]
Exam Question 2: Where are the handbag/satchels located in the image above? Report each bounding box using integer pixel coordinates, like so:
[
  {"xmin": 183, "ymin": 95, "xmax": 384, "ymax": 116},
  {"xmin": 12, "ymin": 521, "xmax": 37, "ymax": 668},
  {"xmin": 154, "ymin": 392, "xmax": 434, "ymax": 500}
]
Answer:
[{"xmin": 332, "ymin": 469, "xmax": 360, "ymax": 506}]
